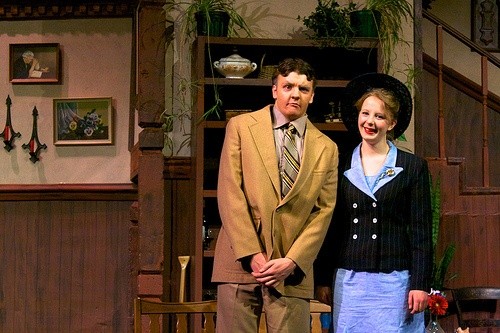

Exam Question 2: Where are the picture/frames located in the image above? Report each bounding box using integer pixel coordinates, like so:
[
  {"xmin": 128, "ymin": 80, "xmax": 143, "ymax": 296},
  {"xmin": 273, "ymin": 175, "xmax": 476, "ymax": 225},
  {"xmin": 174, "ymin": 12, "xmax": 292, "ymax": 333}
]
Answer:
[
  {"xmin": 53, "ymin": 97, "xmax": 115, "ymax": 146},
  {"xmin": 9, "ymin": 42, "xmax": 63, "ymax": 85}
]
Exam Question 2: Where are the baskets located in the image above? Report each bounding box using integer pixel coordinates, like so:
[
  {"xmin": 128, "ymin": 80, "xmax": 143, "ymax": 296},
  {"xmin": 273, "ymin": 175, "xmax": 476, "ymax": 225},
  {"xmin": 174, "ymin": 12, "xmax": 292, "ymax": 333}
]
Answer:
[{"xmin": 257, "ymin": 50, "xmax": 278, "ymax": 78}]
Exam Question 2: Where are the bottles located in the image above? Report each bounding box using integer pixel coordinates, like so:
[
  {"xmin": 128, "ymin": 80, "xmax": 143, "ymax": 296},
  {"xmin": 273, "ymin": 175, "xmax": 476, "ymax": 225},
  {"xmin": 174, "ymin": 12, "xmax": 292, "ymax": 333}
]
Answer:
[{"xmin": 202, "ymin": 216, "xmax": 210, "ymax": 250}]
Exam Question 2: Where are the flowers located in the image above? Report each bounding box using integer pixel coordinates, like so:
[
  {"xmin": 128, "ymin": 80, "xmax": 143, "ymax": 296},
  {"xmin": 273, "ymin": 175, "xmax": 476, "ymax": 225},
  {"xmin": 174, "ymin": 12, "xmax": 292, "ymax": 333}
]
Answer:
[{"xmin": 426, "ymin": 169, "xmax": 461, "ymax": 315}]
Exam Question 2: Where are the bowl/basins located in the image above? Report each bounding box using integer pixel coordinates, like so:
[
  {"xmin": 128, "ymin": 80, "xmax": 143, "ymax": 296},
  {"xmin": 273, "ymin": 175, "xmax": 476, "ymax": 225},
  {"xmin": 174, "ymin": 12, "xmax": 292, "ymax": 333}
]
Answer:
[{"xmin": 213, "ymin": 54, "xmax": 257, "ymax": 79}]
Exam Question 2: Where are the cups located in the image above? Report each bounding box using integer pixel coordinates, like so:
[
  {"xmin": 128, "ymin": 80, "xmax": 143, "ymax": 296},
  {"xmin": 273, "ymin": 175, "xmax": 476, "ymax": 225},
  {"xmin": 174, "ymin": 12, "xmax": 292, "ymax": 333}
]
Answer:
[{"xmin": 324, "ymin": 101, "xmax": 343, "ymax": 124}]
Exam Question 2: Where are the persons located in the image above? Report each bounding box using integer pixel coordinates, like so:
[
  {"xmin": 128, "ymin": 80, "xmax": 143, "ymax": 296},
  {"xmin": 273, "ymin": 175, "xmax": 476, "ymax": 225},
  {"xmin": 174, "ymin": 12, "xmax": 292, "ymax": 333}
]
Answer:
[
  {"xmin": 315, "ymin": 73, "xmax": 434, "ymax": 333},
  {"xmin": 210, "ymin": 57, "xmax": 339, "ymax": 333}
]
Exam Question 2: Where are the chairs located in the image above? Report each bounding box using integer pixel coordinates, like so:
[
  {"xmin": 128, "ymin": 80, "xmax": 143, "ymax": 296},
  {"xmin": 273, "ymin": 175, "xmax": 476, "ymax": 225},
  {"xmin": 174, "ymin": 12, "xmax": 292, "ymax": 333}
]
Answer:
[
  {"xmin": 452, "ymin": 286, "xmax": 500, "ymax": 333},
  {"xmin": 190, "ymin": 36, "xmax": 383, "ymax": 333},
  {"xmin": 134, "ymin": 297, "xmax": 218, "ymax": 333}
]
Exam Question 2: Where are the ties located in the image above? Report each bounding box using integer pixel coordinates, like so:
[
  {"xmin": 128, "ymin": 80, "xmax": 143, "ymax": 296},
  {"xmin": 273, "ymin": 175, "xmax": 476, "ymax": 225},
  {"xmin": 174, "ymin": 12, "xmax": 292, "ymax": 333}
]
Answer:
[{"xmin": 281, "ymin": 123, "xmax": 300, "ymax": 198}]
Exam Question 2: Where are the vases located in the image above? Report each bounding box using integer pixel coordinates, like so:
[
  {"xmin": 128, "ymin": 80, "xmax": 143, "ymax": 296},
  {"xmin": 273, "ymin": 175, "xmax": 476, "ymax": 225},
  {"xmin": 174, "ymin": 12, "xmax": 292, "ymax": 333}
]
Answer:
[{"xmin": 423, "ymin": 311, "xmax": 444, "ymax": 333}]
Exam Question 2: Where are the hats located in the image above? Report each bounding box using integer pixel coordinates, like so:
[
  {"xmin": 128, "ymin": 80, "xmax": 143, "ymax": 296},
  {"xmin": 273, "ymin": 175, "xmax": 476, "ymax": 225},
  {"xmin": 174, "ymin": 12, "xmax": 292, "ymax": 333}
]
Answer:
[{"xmin": 340, "ymin": 72, "xmax": 413, "ymax": 140}]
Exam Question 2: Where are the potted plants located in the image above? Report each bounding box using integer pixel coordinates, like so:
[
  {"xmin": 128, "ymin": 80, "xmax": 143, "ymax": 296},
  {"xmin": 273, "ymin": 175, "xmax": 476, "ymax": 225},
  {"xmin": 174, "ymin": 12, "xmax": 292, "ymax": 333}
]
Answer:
[
  {"xmin": 143, "ymin": 0, "xmax": 256, "ymax": 158},
  {"xmin": 297, "ymin": 0, "xmax": 424, "ymax": 143}
]
